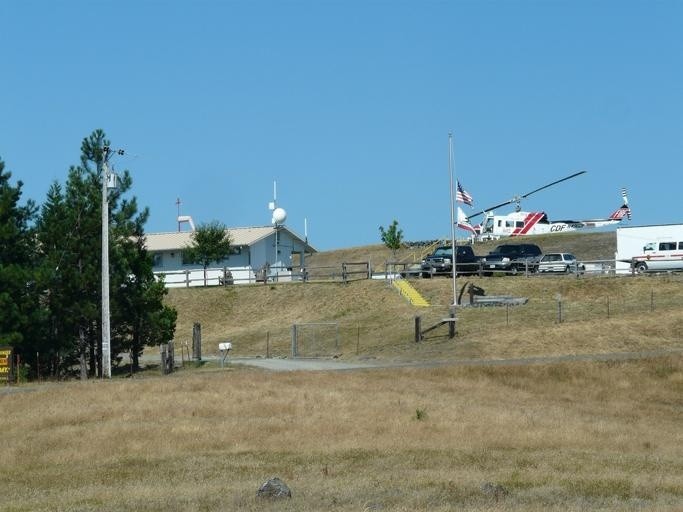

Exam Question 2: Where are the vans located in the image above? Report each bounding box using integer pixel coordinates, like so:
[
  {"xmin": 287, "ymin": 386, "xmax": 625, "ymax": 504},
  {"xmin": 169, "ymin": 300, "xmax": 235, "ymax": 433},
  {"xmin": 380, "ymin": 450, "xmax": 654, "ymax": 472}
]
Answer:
[{"xmin": 630, "ymin": 241, "xmax": 683, "ymax": 274}]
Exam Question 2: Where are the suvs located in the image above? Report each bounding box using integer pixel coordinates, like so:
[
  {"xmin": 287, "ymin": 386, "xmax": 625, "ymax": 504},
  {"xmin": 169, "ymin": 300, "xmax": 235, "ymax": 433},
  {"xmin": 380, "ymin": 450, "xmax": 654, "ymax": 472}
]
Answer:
[{"xmin": 421, "ymin": 244, "xmax": 585, "ymax": 278}]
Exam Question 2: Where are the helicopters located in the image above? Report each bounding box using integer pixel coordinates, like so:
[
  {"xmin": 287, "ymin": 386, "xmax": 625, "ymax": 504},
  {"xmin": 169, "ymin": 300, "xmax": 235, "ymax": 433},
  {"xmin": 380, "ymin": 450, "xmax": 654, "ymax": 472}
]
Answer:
[{"xmin": 454, "ymin": 170, "xmax": 632, "ymax": 243}]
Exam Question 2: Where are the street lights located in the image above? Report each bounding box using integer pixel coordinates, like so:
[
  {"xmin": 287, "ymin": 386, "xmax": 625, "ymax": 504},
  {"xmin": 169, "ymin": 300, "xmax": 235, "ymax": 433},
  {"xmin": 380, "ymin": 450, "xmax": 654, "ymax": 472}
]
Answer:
[{"xmin": 268, "ymin": 201, "xmax": 287, "ymax": 282}]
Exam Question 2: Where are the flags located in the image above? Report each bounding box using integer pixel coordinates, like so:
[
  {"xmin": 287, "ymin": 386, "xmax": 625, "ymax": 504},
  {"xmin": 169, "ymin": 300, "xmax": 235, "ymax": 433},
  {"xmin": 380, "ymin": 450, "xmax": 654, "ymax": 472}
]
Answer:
[
  {"xmin": 457, "ymin": 177, "xmax": 473, "ymax": 206},
  {"xmin": 457, "ymin": 207, "xmax": 477, "ymax": 237}
]
